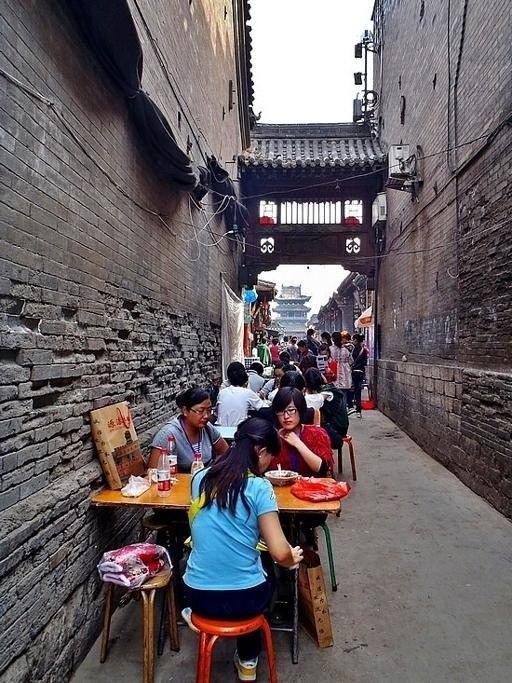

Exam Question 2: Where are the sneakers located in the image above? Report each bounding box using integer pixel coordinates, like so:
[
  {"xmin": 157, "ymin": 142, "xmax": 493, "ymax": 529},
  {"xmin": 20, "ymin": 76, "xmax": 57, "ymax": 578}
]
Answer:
[
  {"xmin": 181, "ymin": 607, "xmax": 201, "ymax": 635},
  {"xmin": 356, "ymin": 412, "xmax": 362, "ymax": 418},
  {"xmin": 347, "ymin": 407, "xmax": 356, "ymax": 415},
  {"xmin": 234, "ymin": 649, "xmax": 259, "ymax": 682},
  {"xmin": 270, "ymin": 603, "xmax": 291, "ymax": 625}
]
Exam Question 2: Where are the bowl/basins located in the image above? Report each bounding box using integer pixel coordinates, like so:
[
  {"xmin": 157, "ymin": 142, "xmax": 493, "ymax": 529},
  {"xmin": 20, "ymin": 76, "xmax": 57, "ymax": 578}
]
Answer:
[
  {"xmin": 266, "ymin": 470, "xmax": 299, "ymax": 485},
  {"xmin": 361, "ymin": 401, "xmax": 374, "ymax": 409}
]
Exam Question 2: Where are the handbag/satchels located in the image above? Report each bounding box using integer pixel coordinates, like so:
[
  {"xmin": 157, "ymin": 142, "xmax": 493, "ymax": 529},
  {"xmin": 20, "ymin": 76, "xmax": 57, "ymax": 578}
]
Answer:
[
  {"xmin": 90, "ymin": 400, "xmax": 147, "ymax": 490},
  {"xmin": 299, "ymin": 547, "xmax": 334, "ymax": 649},
  {"xmin": 326, "ymin": 358, "xmax": 337, "ymax": 381}
]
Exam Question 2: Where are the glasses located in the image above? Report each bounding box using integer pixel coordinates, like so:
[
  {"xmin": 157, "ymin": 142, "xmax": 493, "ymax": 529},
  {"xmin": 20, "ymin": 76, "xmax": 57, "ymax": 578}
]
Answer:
[
  {"xmin": 275, "ymin": 409, "xmax": 297, "ymax": 416},
  {"xmin": 190, "ymin": 408, "xmax": 214, "ymax": 415}
]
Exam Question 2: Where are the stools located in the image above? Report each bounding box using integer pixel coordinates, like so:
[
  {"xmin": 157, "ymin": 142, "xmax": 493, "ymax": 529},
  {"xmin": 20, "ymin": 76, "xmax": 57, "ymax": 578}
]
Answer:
[
  {"xmin": 100, "ymin": 569, "xmax": 179, "ymax": 682},
  {"xmin": 336, "ymin": 436, "xmax": 357, "ymax": 481},
  {"xmin": 139, "ymin": 514, "xmax": 189, "ymax": 561},
  {"xmin": 190, "ymin": 612, "xmax": 277, "ymax": 682},
  {"xmin": 290, "ymin": 512, "xmax": 338, "ymax": 592}
]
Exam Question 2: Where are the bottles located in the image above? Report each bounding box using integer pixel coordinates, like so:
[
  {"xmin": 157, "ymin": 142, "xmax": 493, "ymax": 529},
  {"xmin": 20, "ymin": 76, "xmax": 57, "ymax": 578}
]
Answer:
[
  {"xmin": 157, "ymin": 435, "xmax": 177, "ymax": 496},
  {"xmin": 191, "ymin": 453, "xmax": 203, "ymax": 480}
]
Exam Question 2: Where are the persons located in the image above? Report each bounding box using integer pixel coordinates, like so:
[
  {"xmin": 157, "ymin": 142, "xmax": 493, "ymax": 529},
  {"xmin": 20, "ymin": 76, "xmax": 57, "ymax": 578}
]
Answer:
[
  {"xmin": 217, "ymin": 328, "xmax": 364, "ymax": 448},
  {"xmin": 149, "ymin": 389, "xmax": 229, "ymax": 559},
  {"xmin": 268, "ymin": 387, "xmax": 333, "ymax": 530},
  {"xmin": 182, "ymin": 417, "xmax": 304, "ymax": 682}
]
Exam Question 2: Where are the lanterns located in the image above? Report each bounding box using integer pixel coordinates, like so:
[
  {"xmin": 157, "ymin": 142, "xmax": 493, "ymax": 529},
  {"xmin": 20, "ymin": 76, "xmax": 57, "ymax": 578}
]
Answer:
[
  {"xmin": 260, "ymin": 215, "xmax": 274, "ymax": 225},
  {"xmin": 345, "ymin": 216, "xmax": 358, "ymax": 226}
]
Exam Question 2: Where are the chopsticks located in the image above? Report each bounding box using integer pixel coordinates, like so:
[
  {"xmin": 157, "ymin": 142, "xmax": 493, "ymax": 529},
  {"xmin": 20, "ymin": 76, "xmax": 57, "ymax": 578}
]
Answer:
[{"xmin": 277, "ymin": 462, "xmax": 282, "ymax": 475}]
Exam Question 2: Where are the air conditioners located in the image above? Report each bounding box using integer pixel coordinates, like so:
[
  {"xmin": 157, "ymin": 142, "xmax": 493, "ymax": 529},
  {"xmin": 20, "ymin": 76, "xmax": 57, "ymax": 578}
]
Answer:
[{"xmin": 384, "ymin": 143, "xmax": 412, "ymax": 189}]
